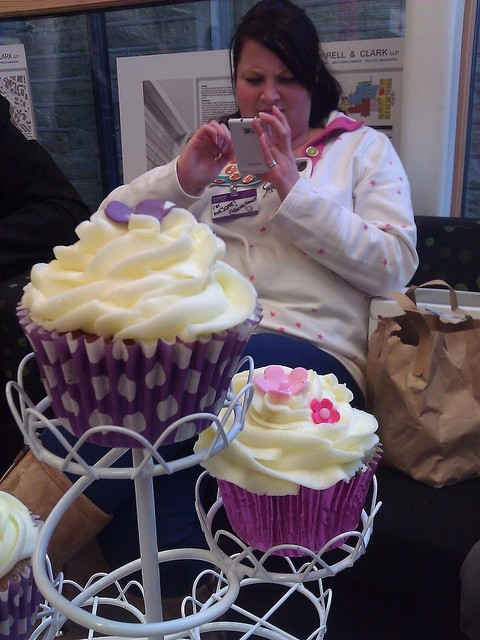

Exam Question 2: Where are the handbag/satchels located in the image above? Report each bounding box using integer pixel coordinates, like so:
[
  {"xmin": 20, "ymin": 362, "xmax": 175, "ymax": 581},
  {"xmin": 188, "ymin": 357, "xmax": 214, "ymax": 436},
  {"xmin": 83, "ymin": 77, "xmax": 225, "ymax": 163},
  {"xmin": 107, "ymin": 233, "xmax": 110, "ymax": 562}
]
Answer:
[{"xmin": 369, "ymin": 279, "xmax": 480, "ymax": 490}]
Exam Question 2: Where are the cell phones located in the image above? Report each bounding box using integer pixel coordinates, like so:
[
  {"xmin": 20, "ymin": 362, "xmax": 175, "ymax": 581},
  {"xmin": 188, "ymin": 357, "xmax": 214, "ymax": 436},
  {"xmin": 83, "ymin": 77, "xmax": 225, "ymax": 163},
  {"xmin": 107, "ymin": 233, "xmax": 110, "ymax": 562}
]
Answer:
[{"xmin": 229, "ymin": 117, "xmax": 272, "ymax": 177}]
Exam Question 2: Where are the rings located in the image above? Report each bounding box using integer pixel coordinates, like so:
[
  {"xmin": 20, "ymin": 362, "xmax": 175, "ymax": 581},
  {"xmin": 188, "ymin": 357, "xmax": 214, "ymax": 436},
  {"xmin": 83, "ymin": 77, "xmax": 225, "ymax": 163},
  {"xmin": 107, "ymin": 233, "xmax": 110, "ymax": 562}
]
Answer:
[{"xmin": 269, "ymin": 160, "xmax": 277, "ymax": 170}]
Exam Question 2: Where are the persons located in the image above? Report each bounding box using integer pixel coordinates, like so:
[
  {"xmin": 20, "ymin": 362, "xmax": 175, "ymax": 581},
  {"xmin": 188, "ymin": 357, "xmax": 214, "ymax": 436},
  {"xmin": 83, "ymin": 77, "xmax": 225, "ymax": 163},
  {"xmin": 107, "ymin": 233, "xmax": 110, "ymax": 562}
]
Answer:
[
  {"xmin": 0, "ymin": 1, "xmax": 420, "ymax": 640},
  {"xmin": 0, "ymin": 88, "xmax": 90, "ymax": 480}
]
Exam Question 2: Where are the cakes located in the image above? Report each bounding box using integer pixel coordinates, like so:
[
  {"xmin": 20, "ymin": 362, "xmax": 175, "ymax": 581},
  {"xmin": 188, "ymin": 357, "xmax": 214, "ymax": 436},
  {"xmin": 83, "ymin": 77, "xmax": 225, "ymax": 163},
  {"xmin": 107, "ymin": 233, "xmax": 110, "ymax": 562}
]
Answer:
[
  {"xmin": 1, "ymin": 492, "xmax": 51, "ymax": 638},
  {"xmin": 13, "ymin": 198, "xmax": 264, "ymax": 447},
  {"xmin": 194, "ymin": 364, "xmax": 384, "ymax": 556}
]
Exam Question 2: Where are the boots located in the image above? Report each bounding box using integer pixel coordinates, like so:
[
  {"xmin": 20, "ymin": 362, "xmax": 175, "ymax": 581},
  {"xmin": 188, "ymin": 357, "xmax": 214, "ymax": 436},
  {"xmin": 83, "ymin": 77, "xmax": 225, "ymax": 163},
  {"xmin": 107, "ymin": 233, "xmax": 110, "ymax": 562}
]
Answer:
[{"xmin": 0, "ymin": 443, "xmax": 114, "ymax": 575}]
